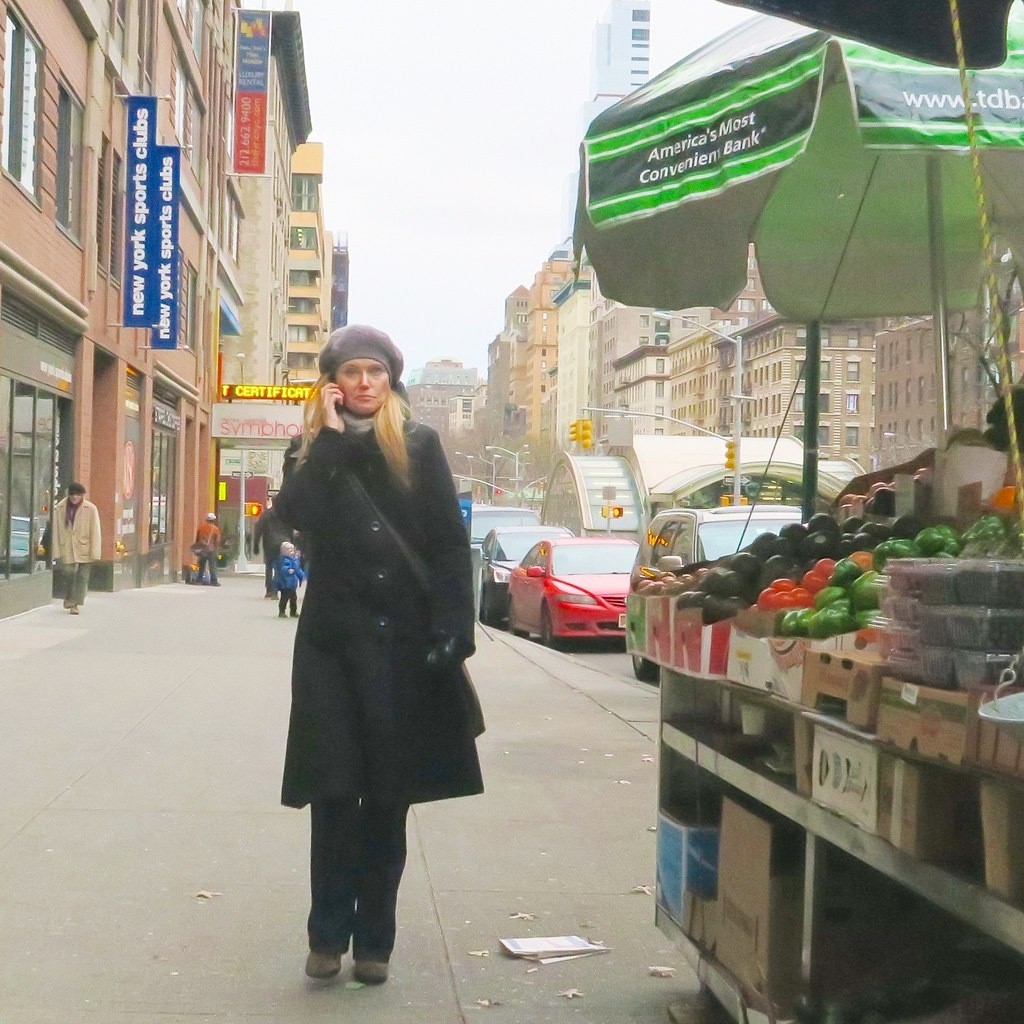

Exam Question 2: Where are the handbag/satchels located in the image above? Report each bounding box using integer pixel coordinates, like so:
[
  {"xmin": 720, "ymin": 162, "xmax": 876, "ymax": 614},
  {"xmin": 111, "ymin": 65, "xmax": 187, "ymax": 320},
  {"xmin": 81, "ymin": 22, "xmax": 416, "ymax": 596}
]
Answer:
[
  {"xmin": 185, "ymin": 567, "xmax": 210, "ymax": 585},
  {"xmin": 190, "ymin": 542, "xmax": 211, "ymax": 557},
  {"xmin": 394, "ymin": 532, "xmax": 486, "ymax": 739}
]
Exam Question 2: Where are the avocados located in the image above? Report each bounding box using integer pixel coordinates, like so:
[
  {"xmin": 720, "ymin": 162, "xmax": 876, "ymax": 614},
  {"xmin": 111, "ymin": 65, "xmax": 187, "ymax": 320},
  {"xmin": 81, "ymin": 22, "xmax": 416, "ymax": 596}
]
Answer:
[
  {"xmin": 659, "ymin": 551, "xmax": 756, "ymax": 626},
  {"xmin": 748, "ymin": 512, "xmax": 940, "ymax": 597}
]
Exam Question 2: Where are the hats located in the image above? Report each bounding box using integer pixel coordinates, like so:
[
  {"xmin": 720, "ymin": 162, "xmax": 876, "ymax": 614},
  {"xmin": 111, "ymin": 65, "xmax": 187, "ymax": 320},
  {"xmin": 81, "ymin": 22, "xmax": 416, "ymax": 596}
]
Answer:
[
  {"xmin": 319, "ymin": 324, "xmax": 404, "ymax": 389},
  {"xmin": 205, "ymin": 513, "xmax": 217, "ymax": 520},
  {"xmin": 281, "ymin": 541, "xmax": 294, "ymax": 553},
  {"xmin": 68, "ymin": 482, "xmax": 86, "ymax": 493}
]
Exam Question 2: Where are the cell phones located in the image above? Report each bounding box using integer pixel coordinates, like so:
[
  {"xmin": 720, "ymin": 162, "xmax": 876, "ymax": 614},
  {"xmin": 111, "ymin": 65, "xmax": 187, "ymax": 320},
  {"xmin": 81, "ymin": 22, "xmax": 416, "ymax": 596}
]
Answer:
[{"xmin": 329, "ymin": 375, "xmax": 344, "ymax": 413}]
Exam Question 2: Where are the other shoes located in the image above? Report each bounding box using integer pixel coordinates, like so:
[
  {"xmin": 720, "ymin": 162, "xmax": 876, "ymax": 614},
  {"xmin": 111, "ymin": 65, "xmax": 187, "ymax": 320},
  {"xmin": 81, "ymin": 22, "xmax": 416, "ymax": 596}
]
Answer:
[
  {"xmin": 211, "ymin": 582, "xmax": 221, "ymax": 586},
  {"xmin": 290, "ymin": 612, "xmax": 300, "ymax": 618},
  {"xmin": 64, "ymin": 600, "xmax": 79, "ymax": 614},
  {"xmin": 355, "ymin": 961, "xmax": 388, "ymax": 984},
  {"xmin": 306, "ymin": 950, "xmax": 340, "ymax": 978},
  {"xmin": 264, "ymin": 589, "xmax": 279, "ymax": 600},
  {"xmin": 278, "ymin": 613, "xmax": 287, "ymax": 618}
]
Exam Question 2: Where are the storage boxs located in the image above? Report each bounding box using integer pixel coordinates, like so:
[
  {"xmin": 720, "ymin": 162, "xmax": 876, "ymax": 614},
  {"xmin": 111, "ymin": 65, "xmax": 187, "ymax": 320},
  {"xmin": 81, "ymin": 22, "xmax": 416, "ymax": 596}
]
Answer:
[{"xmin": 626, "ymin": 557, "xmax": 1024, "ymax": 1024}]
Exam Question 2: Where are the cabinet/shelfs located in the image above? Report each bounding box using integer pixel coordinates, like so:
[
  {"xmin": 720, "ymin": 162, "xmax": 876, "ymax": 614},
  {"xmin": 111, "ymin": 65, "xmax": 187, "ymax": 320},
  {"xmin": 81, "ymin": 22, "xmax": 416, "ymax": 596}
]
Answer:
[{"xmin": 653, "ymin": 663, "xmax": 1024, "ymax": 1023}]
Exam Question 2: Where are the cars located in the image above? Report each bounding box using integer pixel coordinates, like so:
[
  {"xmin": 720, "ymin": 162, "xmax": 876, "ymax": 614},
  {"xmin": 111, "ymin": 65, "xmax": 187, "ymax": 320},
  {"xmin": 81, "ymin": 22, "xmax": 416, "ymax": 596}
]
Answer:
[
  {"xmin": 6, "ymin": 531, "xmax": 31, "ymax": 573},
  {"xmin": 123, "ymin": 498, "xmax": 170, "ymax": 538},
  {"xmin": 38, "ymin": 528, "xmax": 48, "ymax": 557},
  {"xmin": 480, "ymin": 526, "xmax": 576, "ymax": 625},
  {"xmin": 506, "ymin": 538, "xmax": 642, "ymax": 648}
]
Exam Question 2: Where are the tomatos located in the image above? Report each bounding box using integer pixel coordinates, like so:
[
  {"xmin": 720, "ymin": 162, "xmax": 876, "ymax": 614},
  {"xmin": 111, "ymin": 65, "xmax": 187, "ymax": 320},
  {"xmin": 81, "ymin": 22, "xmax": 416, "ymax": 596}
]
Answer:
[{"xmin": 757, "ymin": 550, "xmax": 872, "ymax": 612}]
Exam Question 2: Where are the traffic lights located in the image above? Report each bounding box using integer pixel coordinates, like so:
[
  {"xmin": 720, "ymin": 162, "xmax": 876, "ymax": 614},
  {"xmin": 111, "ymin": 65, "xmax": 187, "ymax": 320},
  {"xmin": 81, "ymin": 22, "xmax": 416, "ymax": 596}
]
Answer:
[
  {"xmin": 719, "ymin": 496, "xmax": 729, "ymax": 506},
  {"xmin": 247, "ymin": 503, "xmax": 263, "ymax": 518},
  {"xmin": 741, "ymin": 497, "xmax": 748, "ymax": 506},
  {"xmin": 569, "ymin": 419, "xmax": 583, "ymax": 442},
  {"xmin": 724, "ymin": 441, "xmax": 736, "ymax": 471},
  {"xmin": 601, "ymin": 507, "xmax": 609, "ymax": 518},
  {"xmin": 582, "ymin": 420, "xmax": 593, "ymax": 449},
  {"xmin": 42, "ymin": 506, "xmax": 46, "ymax": 511},
  {"xmin": 497, "ymin": 490, "xmax": 501, "ymax": 494},
  {"xmin": 613, "ymin": 508, "xmax": 623, "ymax": 519}
]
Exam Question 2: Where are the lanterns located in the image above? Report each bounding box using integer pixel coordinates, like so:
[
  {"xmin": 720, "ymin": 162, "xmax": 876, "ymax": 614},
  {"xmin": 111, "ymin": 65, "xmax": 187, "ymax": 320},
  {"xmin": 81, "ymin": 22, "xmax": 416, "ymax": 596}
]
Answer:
[
  {"xmin": 570, "ymin": 418, "xmax": 592, "ymax": 448},
  {"xmin": 725, "ymin": 442, "xmax": 736, "ymax": 469}
]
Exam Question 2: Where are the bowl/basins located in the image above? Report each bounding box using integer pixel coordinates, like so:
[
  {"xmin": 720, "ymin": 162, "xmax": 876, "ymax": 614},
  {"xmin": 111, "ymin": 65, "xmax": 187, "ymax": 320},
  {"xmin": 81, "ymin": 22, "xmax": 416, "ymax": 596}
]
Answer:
[{"xmin": 978, "ymin": 693, "xmax": 1024, "ymax": 746}]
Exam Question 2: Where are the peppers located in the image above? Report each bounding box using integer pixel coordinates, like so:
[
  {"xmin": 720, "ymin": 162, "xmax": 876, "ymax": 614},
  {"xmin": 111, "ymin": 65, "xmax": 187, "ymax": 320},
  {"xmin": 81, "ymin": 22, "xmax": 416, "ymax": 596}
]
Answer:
[{"xmin": 775, "ymin": 509, "xmax": 1024, "ymax": 639}]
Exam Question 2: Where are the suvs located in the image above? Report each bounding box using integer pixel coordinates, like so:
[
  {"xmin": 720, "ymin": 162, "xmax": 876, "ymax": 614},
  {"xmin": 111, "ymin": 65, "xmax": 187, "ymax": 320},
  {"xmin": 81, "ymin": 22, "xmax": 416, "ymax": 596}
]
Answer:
[
  {"xmin": 624, "ymin": 506, "xmax": 806, "ymax": 682},
  {"xmin": 469, "ymin": 506, "xmax": 542, "ymax": 545}
]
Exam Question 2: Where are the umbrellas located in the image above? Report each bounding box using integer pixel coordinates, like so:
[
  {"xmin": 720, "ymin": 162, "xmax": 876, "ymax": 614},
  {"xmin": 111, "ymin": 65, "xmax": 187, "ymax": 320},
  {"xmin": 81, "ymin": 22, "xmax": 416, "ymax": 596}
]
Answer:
[{"xmin": 573, "ymin": 12, "xmax": 1024, "ymax": 451}]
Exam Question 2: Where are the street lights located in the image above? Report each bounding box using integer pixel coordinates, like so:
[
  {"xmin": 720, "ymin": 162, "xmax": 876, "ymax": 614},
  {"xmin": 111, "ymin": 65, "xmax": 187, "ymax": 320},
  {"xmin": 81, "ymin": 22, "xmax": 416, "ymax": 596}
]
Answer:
[
  {"xmin": 879, "ymin": 429, "xmax": 938, "ymax": 454},
  {"xmin": 485, "ymin": 444, "xmax": 528, "ymax": 489},
  {"xmin": 651, "ymin": 310, "xmax": 743, "ymax": 509},
  {"xmin": 465, "ymin": 454, "xmax": 499, "ymax": 504},
  {"xmin": 454, "ymin": 450, "xmax": 473, "ymax": 478},
  {"xmin": 494, "ymin": 452, "xmax": 526, "ymax": 491}
]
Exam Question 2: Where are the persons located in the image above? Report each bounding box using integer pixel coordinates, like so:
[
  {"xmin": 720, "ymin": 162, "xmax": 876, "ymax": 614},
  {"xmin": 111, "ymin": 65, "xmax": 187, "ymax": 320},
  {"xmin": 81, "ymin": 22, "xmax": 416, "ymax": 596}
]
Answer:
[
  {"xmin": 272, "ymin": 322, "xmax": 486, "ymax": 984},
  {"xmin": 52, "ymin": 482, "xmax": 102, "ymax": 614},
  {"xmin": 253, "ymin": 494, "xmax": 294, "ymax": 600},
  {"xmin": 271, "ymin": 541, "xmax": 305, "ymax": 619},
  {"xmin": 195, "ymin": 513, "xmax": 221, "ymax": 587}
]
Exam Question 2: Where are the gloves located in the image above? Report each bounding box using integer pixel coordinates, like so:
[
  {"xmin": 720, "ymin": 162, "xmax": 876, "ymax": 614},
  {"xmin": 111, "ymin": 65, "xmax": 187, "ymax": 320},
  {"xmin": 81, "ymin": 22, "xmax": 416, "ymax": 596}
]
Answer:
[
  {"xmin": 425, "ymin": 636, "xmax": 476, "ymax": 675},
  {"xmin": 253, "ymin": 545, "xmax": 259, "ymax": 555}
]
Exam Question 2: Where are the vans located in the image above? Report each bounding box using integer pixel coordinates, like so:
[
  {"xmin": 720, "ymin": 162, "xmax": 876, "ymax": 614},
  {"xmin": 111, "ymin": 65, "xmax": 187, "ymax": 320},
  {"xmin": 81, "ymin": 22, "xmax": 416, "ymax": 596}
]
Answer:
[{"xmin": 11, "ymin": 516, "xmax": 40, "ymax": 549}]
